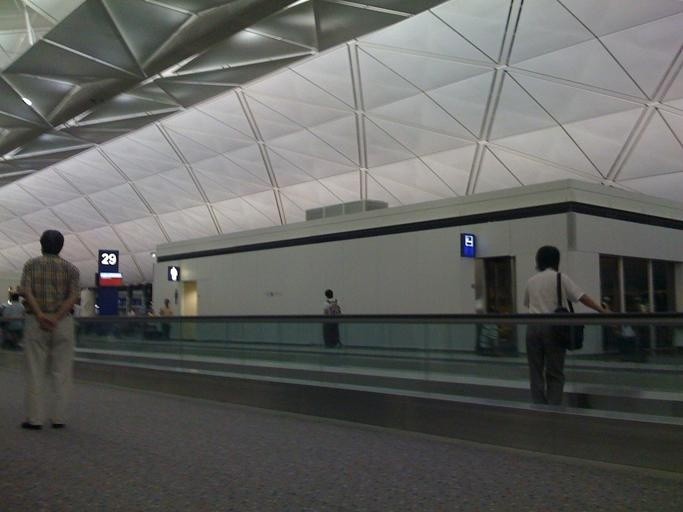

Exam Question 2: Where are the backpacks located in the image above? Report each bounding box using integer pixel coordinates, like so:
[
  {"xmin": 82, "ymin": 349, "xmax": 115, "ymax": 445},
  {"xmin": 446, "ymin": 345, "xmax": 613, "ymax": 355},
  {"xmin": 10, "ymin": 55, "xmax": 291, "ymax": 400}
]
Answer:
[{"xmin": 326, "ymin": 299, "xmax": 341, "ymax": 316}]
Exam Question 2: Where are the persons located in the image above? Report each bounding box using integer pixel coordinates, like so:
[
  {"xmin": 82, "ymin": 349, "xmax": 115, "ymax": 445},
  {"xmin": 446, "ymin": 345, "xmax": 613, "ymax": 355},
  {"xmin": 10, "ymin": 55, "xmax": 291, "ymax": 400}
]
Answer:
[
  {"xmin": 321, "ymin": 285, "xmax": 343, "ymax": 345},
  {"xmin": 20, "ymin": 230, "xmax": 80, "ymax": 430},
  {"xmin": 520, "ymin": 243, "xmax": 612, "ymax": 407},
  {"xmin": 604, "ymin": 291, "xmax": 658, "ymax": 363},
  {"xmin": 0, "ymin": 288, "xmax": 174, "ymax": 350},
  {"xmin": 478, "ymin": 306, "xmax": 508, "ymax": 356}
]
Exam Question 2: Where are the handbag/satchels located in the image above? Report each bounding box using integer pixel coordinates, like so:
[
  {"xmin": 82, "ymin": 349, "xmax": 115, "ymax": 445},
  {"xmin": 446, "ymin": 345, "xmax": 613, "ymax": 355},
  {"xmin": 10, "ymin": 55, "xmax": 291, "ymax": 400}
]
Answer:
[{"xmin": 553, "ymin": 307, "xmax": 585, "ymax": 350}]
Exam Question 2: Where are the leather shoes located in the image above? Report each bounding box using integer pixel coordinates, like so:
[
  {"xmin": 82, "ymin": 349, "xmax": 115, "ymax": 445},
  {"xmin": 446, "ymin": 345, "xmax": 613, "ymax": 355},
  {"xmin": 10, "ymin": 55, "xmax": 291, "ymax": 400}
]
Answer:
[{"xmin": 21, "ymin": 421, "xmax": 41, "ymax": 429}]
